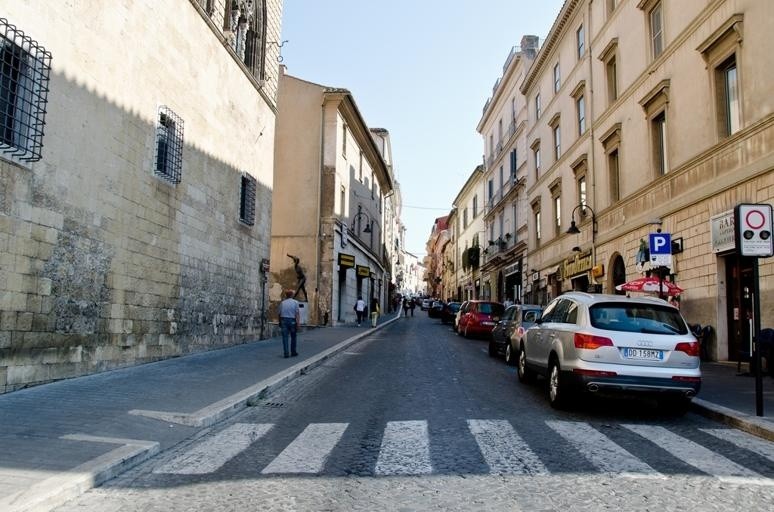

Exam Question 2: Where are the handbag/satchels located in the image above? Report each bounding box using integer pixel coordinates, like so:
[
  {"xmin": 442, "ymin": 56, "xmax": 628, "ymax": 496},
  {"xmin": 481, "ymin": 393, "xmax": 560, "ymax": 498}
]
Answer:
[
  {"xmin": 375, "ymin": 302, "xmax": 381, "ymax": 314},
  {"xmin": 353, "ymin": 300, "xmax": 359, "ymax": 312}
]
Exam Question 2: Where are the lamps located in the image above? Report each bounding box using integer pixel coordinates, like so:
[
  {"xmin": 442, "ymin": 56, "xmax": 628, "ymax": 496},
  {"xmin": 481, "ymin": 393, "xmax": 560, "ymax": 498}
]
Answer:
[
  {"xmin": 565, "ymin": 205, "xmax": 598, "ymax": 243},
  {"xmin": 352, "ymin": 212, "xmax": 372, "ymax": 239}
]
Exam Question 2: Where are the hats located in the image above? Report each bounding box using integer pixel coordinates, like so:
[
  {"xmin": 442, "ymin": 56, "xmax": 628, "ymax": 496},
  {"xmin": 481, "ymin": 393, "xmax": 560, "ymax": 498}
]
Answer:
[{"xmin": 285, "ymin": 289, "xmax": 295, "ymax": 298}]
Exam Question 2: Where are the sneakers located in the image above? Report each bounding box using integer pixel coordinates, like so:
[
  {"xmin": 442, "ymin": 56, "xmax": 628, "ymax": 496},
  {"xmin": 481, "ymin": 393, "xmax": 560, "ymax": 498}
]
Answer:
[{"xmin": 283, "ymin": 351, "xmax": 299, "ymax": 359}]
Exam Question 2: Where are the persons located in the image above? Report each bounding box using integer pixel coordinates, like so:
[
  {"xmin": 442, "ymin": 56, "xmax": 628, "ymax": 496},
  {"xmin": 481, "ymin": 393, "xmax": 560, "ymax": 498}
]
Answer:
[
  {"xmin": 503, "ymin": 296, "xmax": 514, "ymax": 309},
  {"xmin": 409, "ymin": 299, "xmax": 414, "ymax": 316},
  {"xmin": 402, "ymin": 295, "xmax": 408, "ymax": 317},
  {"xmin": 389, "ymin": 293, "xmax": 401, "ymax": 312},
  {"xmin": 370, "ymin": 297, "xmax": 379, "ymax": 328},
  {"xmin": 355, "ymin": 296, "xmax": 366, "ymax": 327},
  {"xmin": 513, "ymin": 299, "xmax": 519, "ymax": 305},
  {"xmin": 278, "ymin": 288, "xmax": 299, "ymax": 358}
]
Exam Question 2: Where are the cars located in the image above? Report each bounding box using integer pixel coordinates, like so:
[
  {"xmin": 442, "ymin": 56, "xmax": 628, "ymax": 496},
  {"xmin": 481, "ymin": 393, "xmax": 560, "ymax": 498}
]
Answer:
[{"xmin": 410, "ymin": 291, "xmax": 702, "ymax": 410}]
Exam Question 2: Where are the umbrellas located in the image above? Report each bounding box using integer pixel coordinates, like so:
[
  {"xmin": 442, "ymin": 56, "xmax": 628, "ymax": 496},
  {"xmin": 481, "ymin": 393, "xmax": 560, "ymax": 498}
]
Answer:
[{"xmin": 614, "ymin": 275, "xmax": 684, "ymax": 297}]
